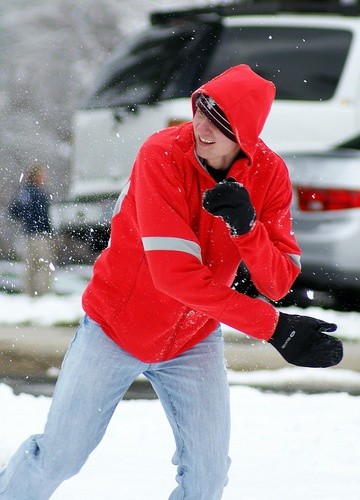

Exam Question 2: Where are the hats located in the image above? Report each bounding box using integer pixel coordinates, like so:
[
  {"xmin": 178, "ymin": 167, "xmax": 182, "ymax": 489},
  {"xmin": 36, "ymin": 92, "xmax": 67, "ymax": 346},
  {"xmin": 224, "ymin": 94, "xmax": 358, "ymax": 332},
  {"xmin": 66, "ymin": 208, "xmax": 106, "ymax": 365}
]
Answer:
[{"xmin": 196, "ymin": 94, "xmax": 238, "ymax": 144}]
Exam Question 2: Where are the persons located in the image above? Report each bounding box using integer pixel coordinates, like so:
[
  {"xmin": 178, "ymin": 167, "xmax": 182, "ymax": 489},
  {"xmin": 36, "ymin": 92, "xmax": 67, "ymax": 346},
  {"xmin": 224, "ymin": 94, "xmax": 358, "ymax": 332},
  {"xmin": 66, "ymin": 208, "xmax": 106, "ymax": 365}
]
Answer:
[
  {"xmin": 0, "ymin": 62, "xmax": 344, "ymax": 500},
  {"xmin": 6, "ymin": 162, "xmax": 58, "ymax": 296}
]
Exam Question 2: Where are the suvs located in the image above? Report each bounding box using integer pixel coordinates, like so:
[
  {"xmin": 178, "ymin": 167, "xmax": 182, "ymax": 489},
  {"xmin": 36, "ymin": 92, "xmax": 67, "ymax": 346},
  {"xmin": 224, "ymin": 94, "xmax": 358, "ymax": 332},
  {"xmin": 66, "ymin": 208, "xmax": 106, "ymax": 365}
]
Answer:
[{"xmin": 48, "ymin": 2, "xmax": 359, "ymax": 310}]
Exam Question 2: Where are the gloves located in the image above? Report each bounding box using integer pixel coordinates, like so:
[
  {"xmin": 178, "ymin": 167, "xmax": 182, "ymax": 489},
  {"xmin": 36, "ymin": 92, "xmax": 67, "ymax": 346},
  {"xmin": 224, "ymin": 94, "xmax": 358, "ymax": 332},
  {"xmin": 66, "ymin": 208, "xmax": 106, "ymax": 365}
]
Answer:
[
  {"xmin": 202, "ymin": 177, "xmax": 256, "ymax": 235},
  {"xmin": 267, "ymin": 312, "xmax": 343, "ymax": 368}
]
